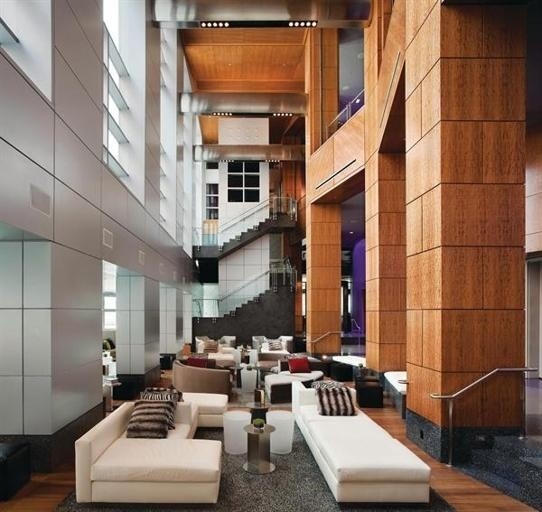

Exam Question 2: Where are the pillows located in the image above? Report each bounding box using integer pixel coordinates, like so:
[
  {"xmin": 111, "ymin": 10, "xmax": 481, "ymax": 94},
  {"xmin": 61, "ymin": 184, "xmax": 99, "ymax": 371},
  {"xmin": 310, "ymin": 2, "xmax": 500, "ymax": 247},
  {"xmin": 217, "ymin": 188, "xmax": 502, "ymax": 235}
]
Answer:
[
  {"xmin": 127, "ymin": 399, "xmax": 174, "ymax": 439},
  {"xmin": 268, "ymin": 341, "xmax": 282, "ymax": 351},
  {"xmin": 140, "ymin": 391, "xmax": 179, "ymax": 430},
  {"xmin": 288, "ymin": 357, "xmax": 311, "ymax": 373},
  {"xmin": 187, "ymin": 357, "xmax": 208, "ymax": 368},
  {"xmin": 315, "ymin": 386, "xmax": 355, "ymax": 416}
]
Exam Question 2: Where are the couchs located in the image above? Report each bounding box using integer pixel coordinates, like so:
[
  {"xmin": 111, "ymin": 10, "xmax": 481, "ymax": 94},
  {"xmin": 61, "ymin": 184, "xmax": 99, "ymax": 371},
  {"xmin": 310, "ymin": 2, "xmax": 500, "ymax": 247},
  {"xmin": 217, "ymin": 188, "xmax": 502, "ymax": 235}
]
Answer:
[
  {"xmin": 218, "ymin": 335, "xmax": 236, "ymax": 348},
  {"xmin": 173, "ymin": 357, "xmax": 230, "ymax": 394},
  {"xmin": 252, "ymin": 336, "xmax": 267, "ymax": 352},
  {"xmin": 195, "ymin": 335, "xmax": 217, "ymax": 354},
  {"xmin": 278, "ymin": 335, "xmax": 293, "ymax": 353},
  {"xmin": 75, "ymin": 393, "xmax": 228, "ymax": 505},
  {"xmin": 290, "ymin": 380, "xmax": 431, "ymax": 503},
  {"xmin": 264, "ymin": 359, "xmax": 324, "ymax": 404}
]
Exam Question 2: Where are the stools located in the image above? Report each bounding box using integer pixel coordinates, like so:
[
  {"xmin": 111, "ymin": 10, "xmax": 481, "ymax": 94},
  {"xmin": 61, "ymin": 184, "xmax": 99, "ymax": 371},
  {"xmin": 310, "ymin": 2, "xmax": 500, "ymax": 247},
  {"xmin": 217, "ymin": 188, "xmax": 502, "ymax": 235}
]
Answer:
[
  {"xmin": 357, "ymin": 382, "xmax": 383, "ymax": 408},
  {"xmin": 330, "ymin": 363, "xmax": 353, "ymax": 381},
  {"xmin": 355, "ymin": 375, "xmax": 377, "ymax": 389}
]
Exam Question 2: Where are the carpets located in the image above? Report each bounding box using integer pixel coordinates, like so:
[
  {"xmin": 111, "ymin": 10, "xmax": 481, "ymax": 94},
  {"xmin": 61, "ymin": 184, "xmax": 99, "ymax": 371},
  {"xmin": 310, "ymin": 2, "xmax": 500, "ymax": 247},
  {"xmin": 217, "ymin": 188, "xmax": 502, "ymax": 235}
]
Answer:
[{"xmin": 54, "ymin": 413, "xmax": 456, "ymax": 511}]
